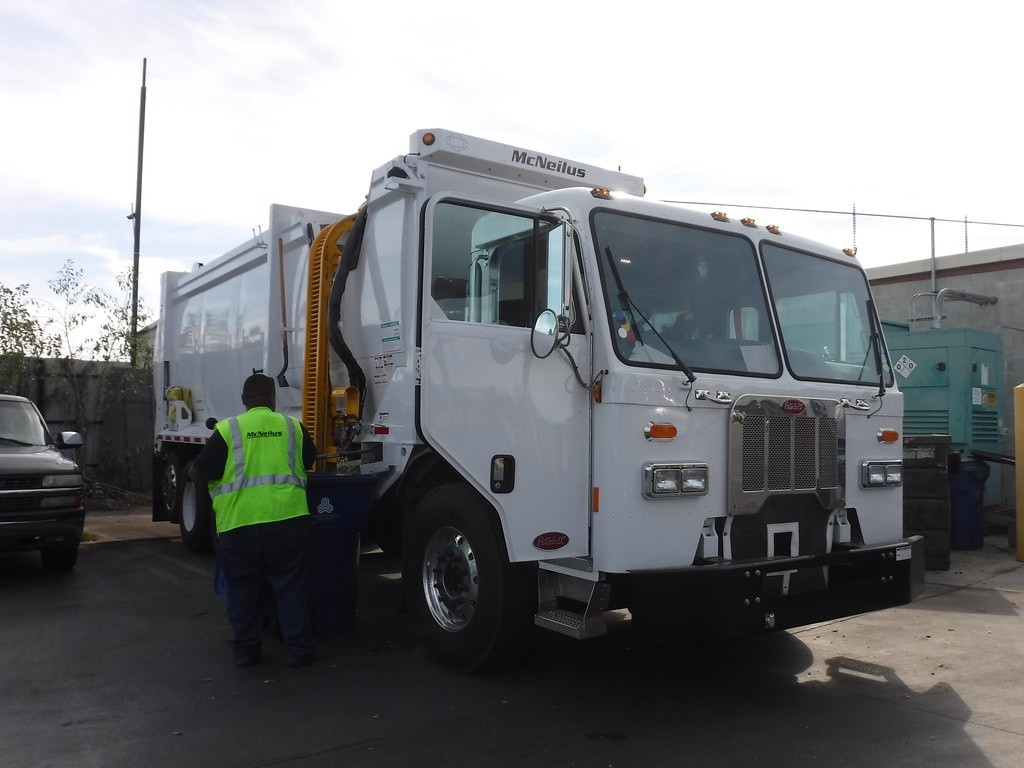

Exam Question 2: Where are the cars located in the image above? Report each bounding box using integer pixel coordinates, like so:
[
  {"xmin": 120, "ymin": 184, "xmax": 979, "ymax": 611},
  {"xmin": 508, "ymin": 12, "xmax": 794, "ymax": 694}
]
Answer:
[{"xmin": 0, "ymin": 394, "xmax": 90, "ymax": 577}]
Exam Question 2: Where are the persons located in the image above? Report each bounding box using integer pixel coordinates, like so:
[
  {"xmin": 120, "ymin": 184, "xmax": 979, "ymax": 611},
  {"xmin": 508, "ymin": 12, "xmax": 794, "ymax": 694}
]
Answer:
[{"xmin": 188, "ymin": 375, "xmax": 317, "ymax": 670}]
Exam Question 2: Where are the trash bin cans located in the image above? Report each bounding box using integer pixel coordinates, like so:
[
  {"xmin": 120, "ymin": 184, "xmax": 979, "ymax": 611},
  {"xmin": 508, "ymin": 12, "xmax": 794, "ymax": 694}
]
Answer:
[{"xmin": 950, "ymin": 459, "xmax": 991, "ymax": 550}]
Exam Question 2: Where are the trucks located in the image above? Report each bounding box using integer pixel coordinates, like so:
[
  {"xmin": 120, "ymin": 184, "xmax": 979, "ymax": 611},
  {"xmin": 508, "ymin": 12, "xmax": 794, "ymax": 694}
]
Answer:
[{"xmin": 149, "ymin": 127, "xmax": 932, "ymax": 668}]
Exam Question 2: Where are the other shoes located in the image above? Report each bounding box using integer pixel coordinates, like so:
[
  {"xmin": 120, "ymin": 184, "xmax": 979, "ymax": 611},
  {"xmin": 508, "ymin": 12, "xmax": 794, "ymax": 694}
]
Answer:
[
  {"xmin": 285, "ymin": 643, "xmax": 319, "ymax": 668},
  {"xmin": 232, "ymin": 644, "xmax": 262, "ymax": 667}
]
